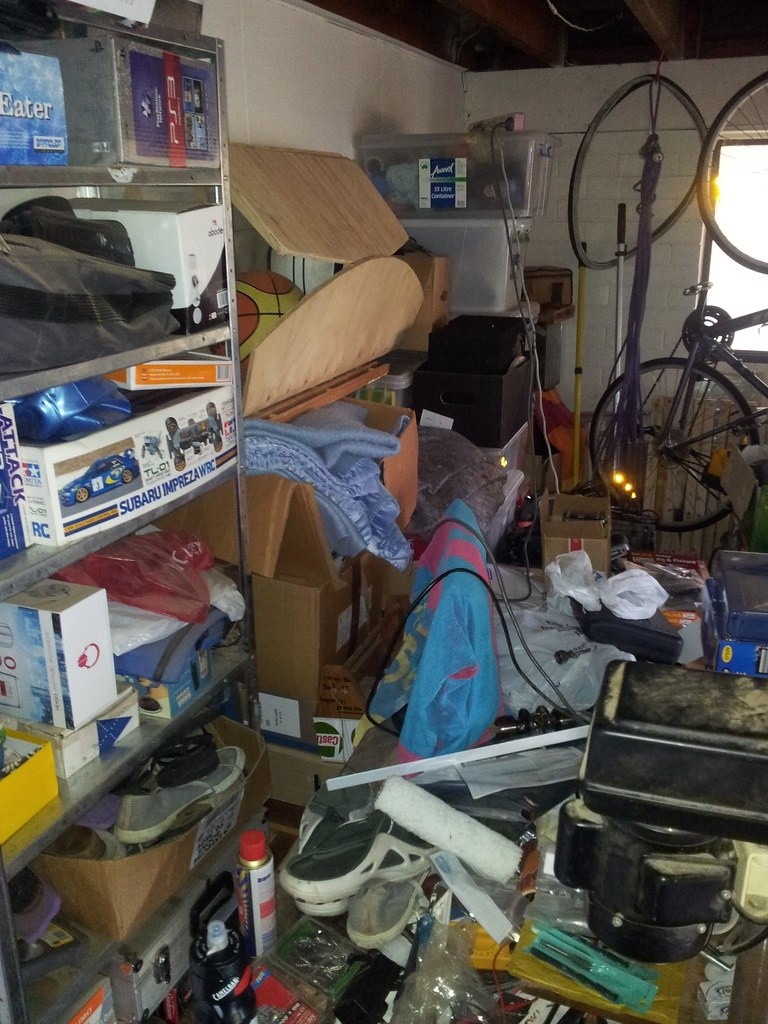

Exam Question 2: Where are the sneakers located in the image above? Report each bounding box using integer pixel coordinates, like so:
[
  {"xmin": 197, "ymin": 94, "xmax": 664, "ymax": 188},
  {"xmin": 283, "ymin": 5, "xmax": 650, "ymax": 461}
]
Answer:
[
  {"xmin": 279, "ymin": 809, "xmax": 438, "ymax": 903},
  {"xmin": 295, "ymin": 894, "xmax": 356, "ymax": 917}
]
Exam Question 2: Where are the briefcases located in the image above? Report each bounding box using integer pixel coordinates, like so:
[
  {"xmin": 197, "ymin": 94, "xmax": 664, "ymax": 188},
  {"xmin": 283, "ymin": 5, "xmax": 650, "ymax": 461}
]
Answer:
[
  {"xmin": 522, "ymin": 265, "xmax": 573, "ymax": 309},
  {"xmin": 705, "ymin": 610, "xmax": 768, "ymax": 677},
  {"xmin": 705, "ymin": 550, "xmax": 768, "ymax": 642}
]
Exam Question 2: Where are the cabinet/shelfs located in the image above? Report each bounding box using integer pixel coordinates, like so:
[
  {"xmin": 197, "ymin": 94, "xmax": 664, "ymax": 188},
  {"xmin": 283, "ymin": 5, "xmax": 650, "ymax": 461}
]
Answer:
[{"xmin": 0, "ymin": 0, "xmax": 276, "ymax": 1024}]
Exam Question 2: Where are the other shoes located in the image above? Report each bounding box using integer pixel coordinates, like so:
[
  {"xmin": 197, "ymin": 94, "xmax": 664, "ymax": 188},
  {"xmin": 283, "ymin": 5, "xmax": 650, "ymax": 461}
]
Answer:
[
  {"xmin": 41, "ymin": 823, "xmax": 128, "ymax": 861},
  {"xmin": 115, "ymin": 745, "xmax": 246, "ymax": 843},
  {"xmin": 346, "ymin": 869, "xmax": 431, "ymax": 949}
]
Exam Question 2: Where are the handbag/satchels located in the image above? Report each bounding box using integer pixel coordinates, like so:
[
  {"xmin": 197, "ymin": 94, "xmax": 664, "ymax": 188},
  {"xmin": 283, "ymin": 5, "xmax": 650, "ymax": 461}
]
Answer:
[
  {"xmin": 0, "ymin": 196, "xmax": 135, "ymax": 267},
  {"xmin": 570, "ymin": 598, "xmax": 683, "ymax": 665},
  {"xmin": 312, "ymin": 717, "xmax": 361, "ymax": 763},
  {"xmin": 114, "ymin": 606, "xmax": 244, "ymax": 695},
  {"xmin": 0, "ymin": 233, "xmax": 180, "ymax": 373}
]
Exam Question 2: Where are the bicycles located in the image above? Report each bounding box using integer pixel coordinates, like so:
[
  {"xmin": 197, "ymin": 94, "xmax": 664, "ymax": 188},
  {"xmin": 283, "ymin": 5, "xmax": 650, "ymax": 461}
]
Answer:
[{"xmin": 587, "ymin": 281, "xmax": 768, "ymax": 534}]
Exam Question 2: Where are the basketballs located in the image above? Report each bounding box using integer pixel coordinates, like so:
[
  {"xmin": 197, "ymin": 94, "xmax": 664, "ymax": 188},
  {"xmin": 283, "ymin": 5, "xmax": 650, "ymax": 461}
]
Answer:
[{"xmin": 211, "ymin": 270, "xmax": 305, "ymax": 378}]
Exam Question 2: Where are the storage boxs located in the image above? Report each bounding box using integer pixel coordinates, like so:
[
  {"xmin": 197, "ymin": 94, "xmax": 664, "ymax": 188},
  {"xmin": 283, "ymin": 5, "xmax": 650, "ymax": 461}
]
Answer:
[
  {"xmin": 70, "ymin": 195, "xmax": 229, "ymax": 334},
  {"xmin": 0, "ymin": 578, "xmax": 117, "ymax": 730},
  {"xmin": 0, "ymin": 727, "xmax": 59, "ymax": 846},
  {"xmin": 359, "ymin": 130, "xmax": 564, "ymax": 217},
  {"xmin": 479, "ymin": 421, "xmax": 529, "ymax": 489},
  {"xmin": 712, "ymin": 549, "xmax": 768, "ymax": 641},
  {"xmin": 701, "ymin": 577, "xmax": 768, "ymax": 678},
  {"xmin": 397, "ymin": 217, "xmax": 536, "ymax": 314},
  {"xmin": 151, "ymin": 472, "xmax": 386, "ymax": 754},
  {"xmin": 627, "ymin": 549, "xmax": 710, "ymax": 581},
  {"xmin": 537, "ymin": 488, "xmax": 614, "ymax": 579},
  {"xmin": 0, "ymin": 50, "xmax": 71, "ymax": 167},
  {"xmin": 15, "ymin": 385, "xmax": 238, "ymax": 547},
  {"xmin": 344, "ymin": 393, "xmax": 420, "ymax": 530},
  {"xmin": 58, "ymin": 974, "xmax": 117, "ymax": 1024},
  {"xmin": 411, "ymin": 355, "xmax": 533, "ymax": 444},
  {"xmin": 534, "ymin": 306, "xmax": 575, "ymax": 393},
  {"xmin": 109, "ymin": 645, "xmax": 214, "ymax": 718},
  {"xmin": 365, "ymin": 351, "xmax": 427, "ymax": 407},
  {"xmin": 0, "ymin": 401, "xmax": 36, "ymax": 561},
  {"xmin": 30, "ymin": 712, "xmax": 272, "ymax": 942},
  {"xmin": 125, "ymin": 818, "xmax": 269, "ymax": 1016},
  {"xmin": 482, "ymin": 470, "xmax": 526, "ymax": 551},
  {"xmin": 19, "ymin": 683, "xmax": 141, "ymax": 779},
  {"xmin": 104, "ymin": 352, "xmax": 233, "ymax": 391},
  {"xmin": 17, "ymin": 35, "xmax": 222, "ymax": 172}
]
